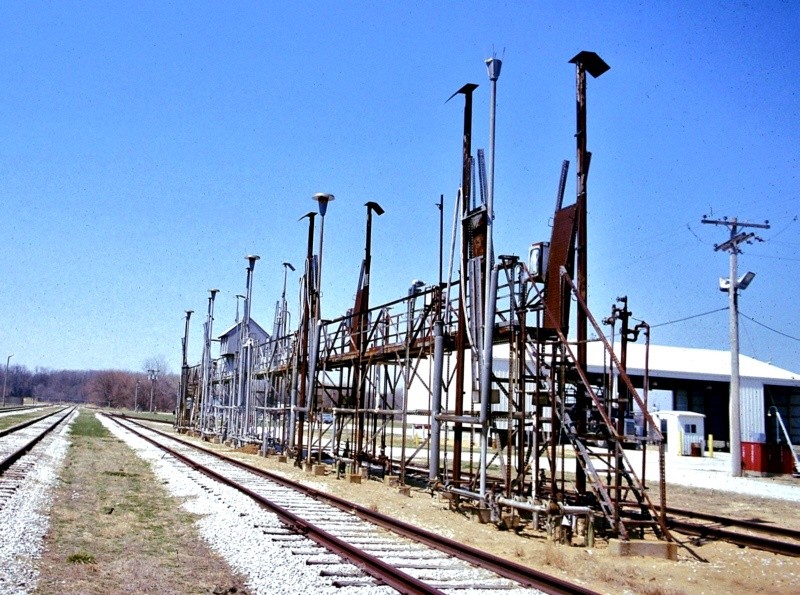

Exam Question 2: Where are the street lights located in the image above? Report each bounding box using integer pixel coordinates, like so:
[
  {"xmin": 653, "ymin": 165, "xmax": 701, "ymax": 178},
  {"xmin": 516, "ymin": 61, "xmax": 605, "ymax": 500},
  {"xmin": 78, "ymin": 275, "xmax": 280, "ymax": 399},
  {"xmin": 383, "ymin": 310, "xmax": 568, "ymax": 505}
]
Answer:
[
  {"xmin": 176, "ymin": 308, "xmax": 195, "ymax": 431},
  {"xmin": 718, "ymin": 271, "xmax": 757, "ymax": 477},
  {"xmin": 201, "ymin": 288, "xmax": 220, "ymax": 432},
  {"xmin": 289, "ymin": 191, "xmax": 337, "ymax": 464},
  {"xmin": 241, "ymin": 254, "xmax": 260, "ymax": 438}
]
